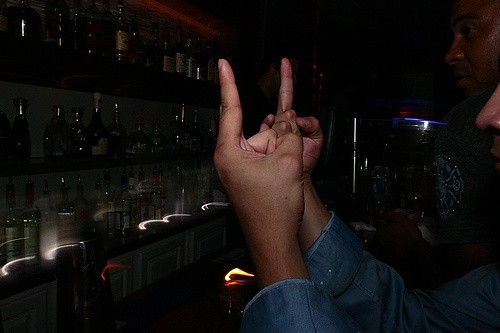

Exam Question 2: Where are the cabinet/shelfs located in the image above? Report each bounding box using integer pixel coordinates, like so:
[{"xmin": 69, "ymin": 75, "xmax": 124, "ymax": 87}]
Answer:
[{"xmin": 0, "ymin": 38, "xmax": 237, "ymax": 333}]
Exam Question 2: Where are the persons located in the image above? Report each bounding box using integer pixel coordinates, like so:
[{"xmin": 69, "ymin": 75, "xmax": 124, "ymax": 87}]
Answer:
[
  {"xmin": 214, "ymin": 58, "xmax": 500, "ymax": 333},
  {"xmin": 243, "ymin": 50, "xmax": 302, "ymax": 130},
  {"xmin": 431, "ymin": 0, "xmax": 500, "ymax": 268}
]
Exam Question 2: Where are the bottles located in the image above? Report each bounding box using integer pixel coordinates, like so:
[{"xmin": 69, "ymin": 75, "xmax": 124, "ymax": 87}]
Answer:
[
  {"xmin": 195, "ymin": 37, "xmax": 224, "ymax": 84},
  {"xmin": 55, "ymin": 175, "xmax": 90, "ymax": 245},
  {"xmin": 107, "ymin": 94, "xmax": 124, "ymax": 156},
  {"xmin": 0, "ymin": 110, "xmax": 12, "ymax": 162},
  {"xmin": 0, "ymin": 0, "xmax": 9, "ymax": 35},
  {"xmin": 176, "ymin": 158, "xmax": 214, "ymax": 212},
  {"xmin": 162, "ymin": 19, "xmax": 176, "ymax": 73},
  {"xmin": 43, "ymin": 104, "xmax": 71, "ymax": 159},
  {"xmin": 69, "ymin": 105, "xmax": 87, "ymax": 155},
  {"xmin": 184, "ymin": 36, "xmax": 197, "ymax": 78},
  {"xmin": 17, "ymin": 0, "xmax": 29, "ymax": 38},
  {"xmin": 130, "ymin": 9, "xmax": 144, "ymax": 63},
  {"xmin": 125, "ymin": 111, "xmax": 166, "ymax": 153},
  {"xmin": 12, "ymin": 96, "xmax": 32, "ymax": 157},
  {"xmin": 94, "ymin": 170, "xmax": 149, "ymax": 239},
  {"xmin": 86, "ymin": 91, "xmax": 108, "ymax": 155},
  {"xmin": 167, "ymin": 102, "xmax": 206, "ymax": 153},
  {"xmin": 176, "ymin": 28, "xmax": 186, "ymax": 76},
  {"xmin": 149, "ymin": 164, "xmax": 176, "ymax": 220},
  {"xmin": 148, "ymin": 21, "xmax": 161, "ymax": 66},
  {"xmin": 19, "ymin": 175, "xmax": 58, "ymax": 265},
  {"xmin": 56, "ymin": 235, "xmax": 117, "ymax": 333},
  {"xmin": 0, "ymin": 174, "xmax": 23, "ymax": 272},
  {"xmin": 115, "ymin": 4, "xmax": 129, "ymax": 62},
  {"xmin": 48, "ymin": 0, "xmax": 116, "ymax": 56}
]
